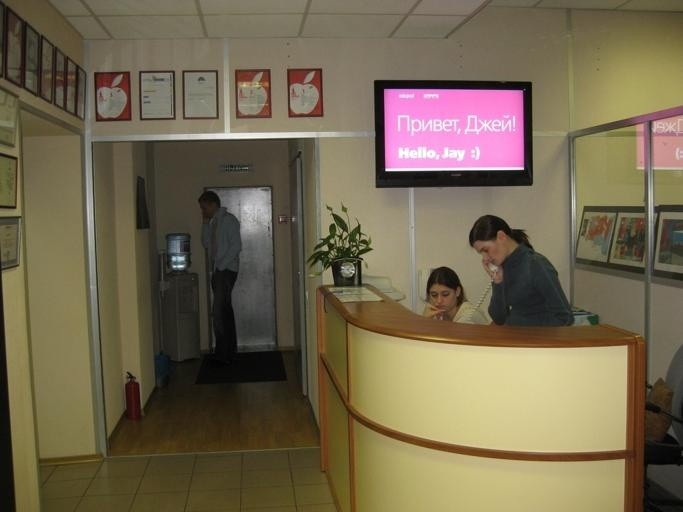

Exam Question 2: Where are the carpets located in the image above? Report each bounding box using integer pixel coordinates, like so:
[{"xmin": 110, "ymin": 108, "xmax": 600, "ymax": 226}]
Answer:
[{"xmin": 195, "ymin": 351, "xmax": 289, "ymax": 385}]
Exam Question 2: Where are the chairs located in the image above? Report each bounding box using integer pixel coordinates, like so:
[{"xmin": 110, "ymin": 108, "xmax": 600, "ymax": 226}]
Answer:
[{"xmin": 642, "ymin": 344, "xmax": 683, "ymax": 512}]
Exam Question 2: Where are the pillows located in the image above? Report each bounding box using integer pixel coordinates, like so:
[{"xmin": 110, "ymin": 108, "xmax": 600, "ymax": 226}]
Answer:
[{"xmin": 644, "ymin": 377, "xmax": 675, "ymax": 446}]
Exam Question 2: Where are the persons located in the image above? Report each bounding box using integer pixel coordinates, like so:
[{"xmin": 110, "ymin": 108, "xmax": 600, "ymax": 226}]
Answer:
[
  {"xmin": 469, "ymin": 214, "xmax": 575, "ymax": 327},
  {"xmin": 199, "ymin": 191, "xmax": 242, "ymax": 367},
  {"xmin": 422, "ymin": 267, "xmax": 488, "ymax": 326}
]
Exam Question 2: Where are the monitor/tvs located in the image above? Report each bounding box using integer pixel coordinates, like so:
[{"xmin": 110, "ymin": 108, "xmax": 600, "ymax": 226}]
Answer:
[{"xmin": 373, "ymin": 79, "xmax": 534, "ymax": 189}]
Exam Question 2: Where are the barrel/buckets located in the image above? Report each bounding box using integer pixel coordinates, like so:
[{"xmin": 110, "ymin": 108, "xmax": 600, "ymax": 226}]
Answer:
[{"xmin": 165, "ymin": 234, "xmax": 191, "ymax": 275}]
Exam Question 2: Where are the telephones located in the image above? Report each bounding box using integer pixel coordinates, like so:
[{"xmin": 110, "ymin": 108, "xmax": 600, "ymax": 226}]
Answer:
[{"xmin": 488, "ymin": 263, "xmax": 499, "ymax": 273}]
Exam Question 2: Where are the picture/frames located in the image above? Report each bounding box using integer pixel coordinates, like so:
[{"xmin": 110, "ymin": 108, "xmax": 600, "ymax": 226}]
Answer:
[
  {"xmin": 652, "ymin": 204, "xmax": 683, "ymax": 280},
  {"xmin": 93, "ymin": 71, "xmax": 132, "ymax": 122},
  {"xmin": 234, "ymin": 69, "xmax": 272, "ymax": 119},
  {"xmin": 286, "ymin": 67, "xmax": 324, "ymax": 118},
  {"xmin": 139, "ymin": 69, "xmax": 176, "ymax": 120},
  {"xmin": 0, "ymin": 151, "xmax": 19, "ymax": 209},
  {"xmin": 607, "ymin": 206, "xmax": 658, "ymax": 274},
  {"xmin": 183, "ymin": 70, "xmax": 219, "ymax": 120},
  {"xmin": 0, "ymin": 216, "xmax": 23, "ymax": 271},
  {"xmin": 0, "ymin": 1, "xmax": 87, "ymax": 147},
  {"xmin": 574, "ymin": 204, "xmax": 617, "ymax": 267}
]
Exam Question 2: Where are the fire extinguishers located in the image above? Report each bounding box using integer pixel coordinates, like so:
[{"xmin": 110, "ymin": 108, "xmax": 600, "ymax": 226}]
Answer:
[{"xmin": 125, "ymin": 371, "xmax": 141, "ymax": 420}]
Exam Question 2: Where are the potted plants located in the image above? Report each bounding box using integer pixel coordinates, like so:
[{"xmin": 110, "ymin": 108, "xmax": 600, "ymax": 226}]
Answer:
[{"xmin": 305, "ymin": 200, "xmax": 374, "ymax": 287}]
